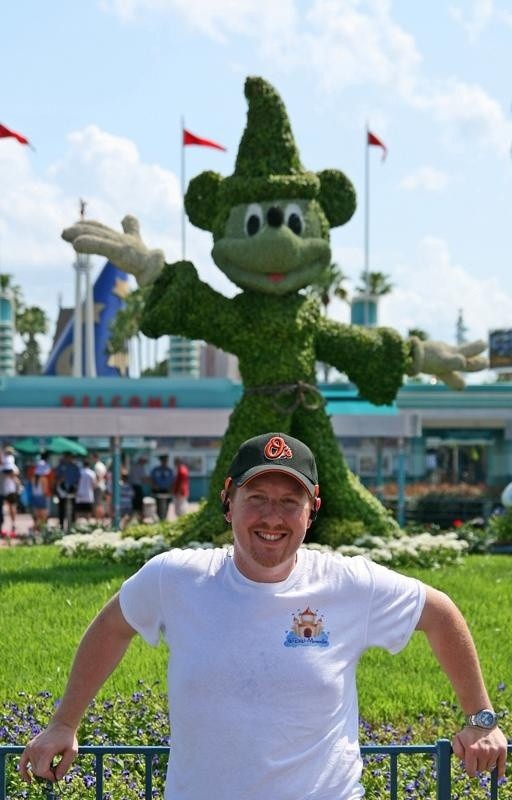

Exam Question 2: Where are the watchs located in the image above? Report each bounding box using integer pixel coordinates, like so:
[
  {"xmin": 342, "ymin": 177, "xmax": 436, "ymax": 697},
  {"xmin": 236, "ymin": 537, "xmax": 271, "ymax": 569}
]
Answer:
[{"xmin": 465, "ymin": 709, "xmax": 497, "ymax": 731}]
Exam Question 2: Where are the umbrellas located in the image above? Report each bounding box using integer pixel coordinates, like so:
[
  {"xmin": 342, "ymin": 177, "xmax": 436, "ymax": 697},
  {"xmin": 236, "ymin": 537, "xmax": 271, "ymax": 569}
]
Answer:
[{"xmin": 13, "ymin": 436, "xmax": 88, "ymax": 457}]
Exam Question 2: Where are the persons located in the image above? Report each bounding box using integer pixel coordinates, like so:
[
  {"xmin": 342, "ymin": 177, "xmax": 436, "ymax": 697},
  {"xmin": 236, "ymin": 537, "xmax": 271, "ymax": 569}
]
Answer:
[
  {"xmin": 0, "ymin": 447, "xmax": 190, "ymax": 540},
  {"xmin": 18, "ymin": 431, "xmax": 508, "ymax": 800}
]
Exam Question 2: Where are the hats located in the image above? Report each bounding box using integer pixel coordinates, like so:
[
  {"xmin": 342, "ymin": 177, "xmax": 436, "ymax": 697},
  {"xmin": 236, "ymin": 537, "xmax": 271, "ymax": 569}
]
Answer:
[{"xmin": 230, "ymin": 432, "xmax": 318, "ymax": 497}]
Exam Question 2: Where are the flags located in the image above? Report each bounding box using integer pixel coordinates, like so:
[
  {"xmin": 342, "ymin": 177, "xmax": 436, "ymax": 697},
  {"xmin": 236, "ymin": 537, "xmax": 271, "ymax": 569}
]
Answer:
[
  {"xmin": 0, "ymin": 124, "xmax": 28, "ymax": 145},
  {"xmin": 369, "ymin": 133, "xmax": 387, "ymax": 160},
  {"xmin": 184, "ymin": 130, "xmax": 225, "ymax": 151}
]
